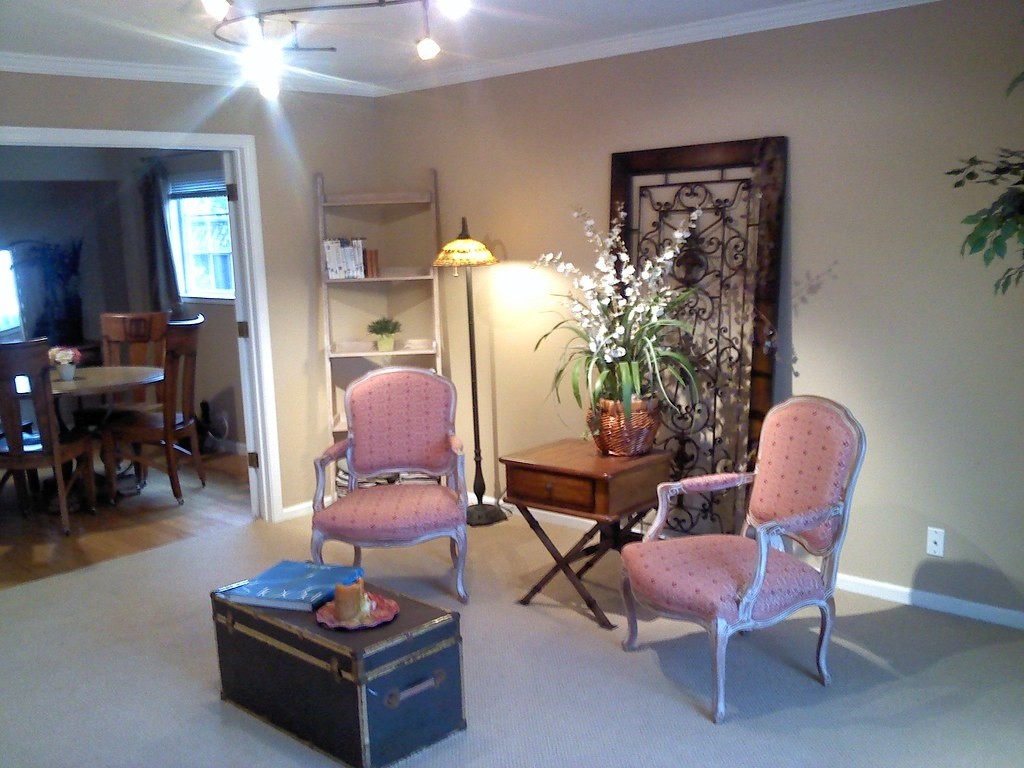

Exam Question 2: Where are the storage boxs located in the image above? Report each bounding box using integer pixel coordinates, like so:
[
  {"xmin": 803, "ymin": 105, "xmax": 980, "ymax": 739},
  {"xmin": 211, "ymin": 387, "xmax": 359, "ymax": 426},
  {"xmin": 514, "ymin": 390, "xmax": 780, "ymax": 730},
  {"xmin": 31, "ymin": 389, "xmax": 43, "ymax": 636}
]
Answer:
[{"xmin": 211, "ymin": 572, "xmax": 469, "ymax": 768}]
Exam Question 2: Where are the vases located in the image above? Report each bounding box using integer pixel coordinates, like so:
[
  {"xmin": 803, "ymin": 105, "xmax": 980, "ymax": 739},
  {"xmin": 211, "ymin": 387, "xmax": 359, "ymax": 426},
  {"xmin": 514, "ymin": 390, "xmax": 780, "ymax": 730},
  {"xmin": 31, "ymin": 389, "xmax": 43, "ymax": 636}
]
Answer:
[
  {"xmin": 55, "ymin": 364, "xmax": 76, "ymax": 381},
  {"xmin": 587, "ymin": 395, "xmax": 661, "ymax": 458}
]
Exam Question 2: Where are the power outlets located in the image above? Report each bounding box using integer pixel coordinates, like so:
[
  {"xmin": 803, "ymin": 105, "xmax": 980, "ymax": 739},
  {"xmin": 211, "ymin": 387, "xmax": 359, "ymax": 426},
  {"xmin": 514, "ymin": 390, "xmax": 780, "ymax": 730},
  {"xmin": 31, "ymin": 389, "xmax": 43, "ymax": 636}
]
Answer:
[{"xmin": 926, "ymin": 526, "xmax": 945, "ymax": 557}]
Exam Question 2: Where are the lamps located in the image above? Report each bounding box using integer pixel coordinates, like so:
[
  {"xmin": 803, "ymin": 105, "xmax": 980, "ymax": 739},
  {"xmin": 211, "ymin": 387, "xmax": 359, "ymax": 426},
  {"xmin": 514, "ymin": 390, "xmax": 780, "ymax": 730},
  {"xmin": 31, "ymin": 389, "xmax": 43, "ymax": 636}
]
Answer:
[{"xmin": 432, "ymin": 216, "xmax": 506, "ymax": 527}]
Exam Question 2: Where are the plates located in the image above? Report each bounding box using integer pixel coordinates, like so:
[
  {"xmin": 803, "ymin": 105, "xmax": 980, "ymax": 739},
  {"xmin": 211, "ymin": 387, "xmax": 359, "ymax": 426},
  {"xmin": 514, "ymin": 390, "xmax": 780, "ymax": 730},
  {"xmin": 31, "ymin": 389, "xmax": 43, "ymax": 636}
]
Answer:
[{"xmin": 316, "ymin": 593, "xmax": 400, "ymax": 629}]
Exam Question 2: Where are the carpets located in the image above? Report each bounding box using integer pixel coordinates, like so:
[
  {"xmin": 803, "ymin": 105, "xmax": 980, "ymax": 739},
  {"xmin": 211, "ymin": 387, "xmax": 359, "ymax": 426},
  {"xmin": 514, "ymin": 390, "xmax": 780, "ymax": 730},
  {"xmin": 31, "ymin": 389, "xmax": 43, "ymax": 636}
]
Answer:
[{"xmin": 1, "ymin": 503, "xmax": 1019, "ymax": 768}]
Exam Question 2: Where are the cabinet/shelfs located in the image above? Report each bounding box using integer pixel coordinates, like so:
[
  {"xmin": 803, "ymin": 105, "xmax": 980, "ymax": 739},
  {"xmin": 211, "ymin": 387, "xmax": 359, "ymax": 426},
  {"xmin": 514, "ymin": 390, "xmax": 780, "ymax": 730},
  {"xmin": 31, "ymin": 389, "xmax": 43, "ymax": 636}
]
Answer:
[{"xmin": 321, "ymin": 196, "xmax": 437, "ymax": 438}]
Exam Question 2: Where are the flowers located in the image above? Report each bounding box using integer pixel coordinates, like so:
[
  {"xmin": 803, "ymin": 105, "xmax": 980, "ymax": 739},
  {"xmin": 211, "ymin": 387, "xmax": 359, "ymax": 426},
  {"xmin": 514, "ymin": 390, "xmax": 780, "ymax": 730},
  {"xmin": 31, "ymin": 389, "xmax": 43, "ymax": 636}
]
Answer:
[
  {"xmin": 48, "ymin": 345, "xmax": 81, "ymax": 367},
  {"xmin": 527, "ymin": 196, "xmax": 719, "ymax": 423}
]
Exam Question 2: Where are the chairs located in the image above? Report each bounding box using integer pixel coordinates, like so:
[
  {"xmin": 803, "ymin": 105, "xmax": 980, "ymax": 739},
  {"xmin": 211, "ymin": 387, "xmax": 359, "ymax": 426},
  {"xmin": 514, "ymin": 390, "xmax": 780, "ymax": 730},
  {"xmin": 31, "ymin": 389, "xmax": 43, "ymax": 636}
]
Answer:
[
  {"xmin": 619, "ymin": 394, "xmax": 866, "ymax": 723},
  {"xmin": 0, "ymin": 337, "xmax": 96, "ymax": 536},
  {"xmin": 100, "ymin": 313, "xmax": 167, "ymax": 410},
  {"xmin": 104, "ymin": 314, "xmax": 206, "ymax": 506},
  {"xmin": 309, "ymin": 367, "xmax": 470, "ymax": 604}
]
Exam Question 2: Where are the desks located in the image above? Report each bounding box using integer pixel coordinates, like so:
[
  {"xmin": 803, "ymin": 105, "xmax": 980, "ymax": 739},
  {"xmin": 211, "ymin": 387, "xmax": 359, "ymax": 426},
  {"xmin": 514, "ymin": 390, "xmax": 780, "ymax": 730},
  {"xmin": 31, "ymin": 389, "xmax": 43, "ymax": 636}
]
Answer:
[
  {"xmin": 7, "ymin": 366, "xmax": 165, "ymax": 511},
  {"xmin": 500, "ymin": 438, "xmax": 676, "ymax": 631}
]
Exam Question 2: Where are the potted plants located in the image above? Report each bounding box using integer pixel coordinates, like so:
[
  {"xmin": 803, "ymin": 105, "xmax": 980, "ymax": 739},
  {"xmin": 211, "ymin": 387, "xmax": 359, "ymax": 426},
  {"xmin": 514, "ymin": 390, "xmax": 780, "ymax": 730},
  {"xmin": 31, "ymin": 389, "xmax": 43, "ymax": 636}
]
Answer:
[{"xmin": 367, "ymin": 316, "xmax": 401, "ymax": 353}]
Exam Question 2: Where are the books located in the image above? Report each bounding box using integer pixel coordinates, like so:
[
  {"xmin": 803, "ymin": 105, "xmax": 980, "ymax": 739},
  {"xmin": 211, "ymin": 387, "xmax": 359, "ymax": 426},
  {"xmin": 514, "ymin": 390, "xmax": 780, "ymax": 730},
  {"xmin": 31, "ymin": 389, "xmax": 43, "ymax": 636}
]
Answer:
[
  {"xmin": 324, "ymin": 238, "xmax": 379, "ymax": 280},
  {"xmin": 336, "ymin": 459, "xmax": 441, "ymax": 498},
  {"xmin": 227, "ymin": 559, "xmax": 364, "ymax": 612}
]
